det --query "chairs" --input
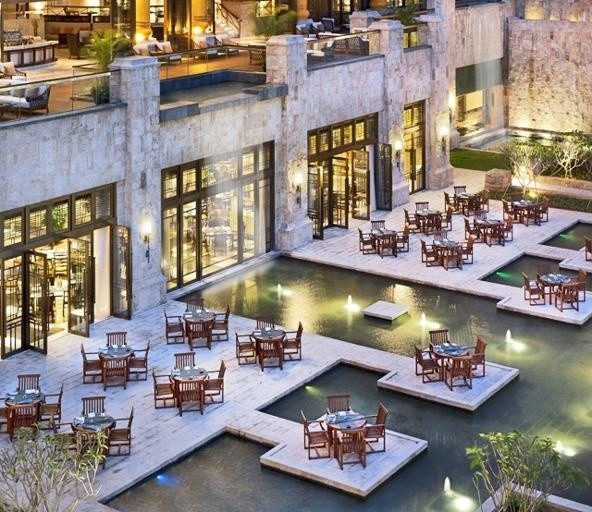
[133,31,240,64]
[296,17,350,41]
[44,24,94,59]
[357,186,592,312]
[248,45,266,68]
[6,250,81,323]
[0,62,52,121]
